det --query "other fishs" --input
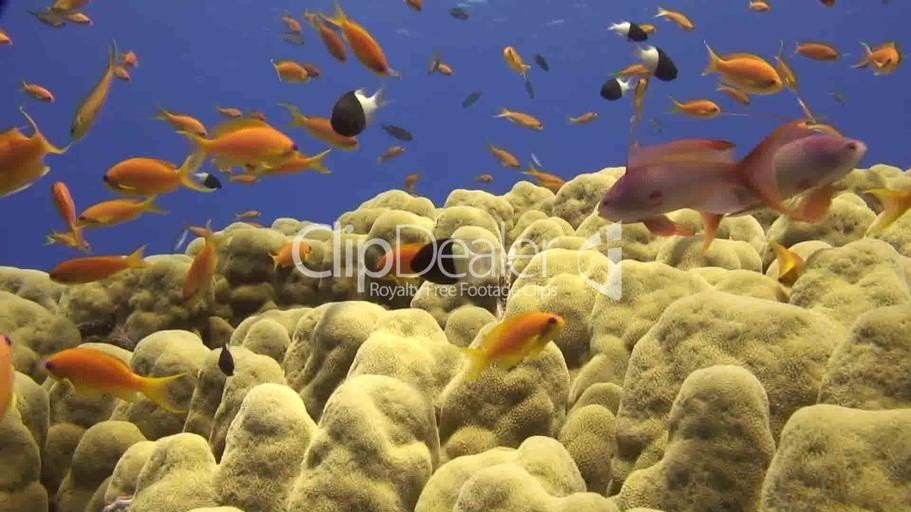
[1,1,907,425]
